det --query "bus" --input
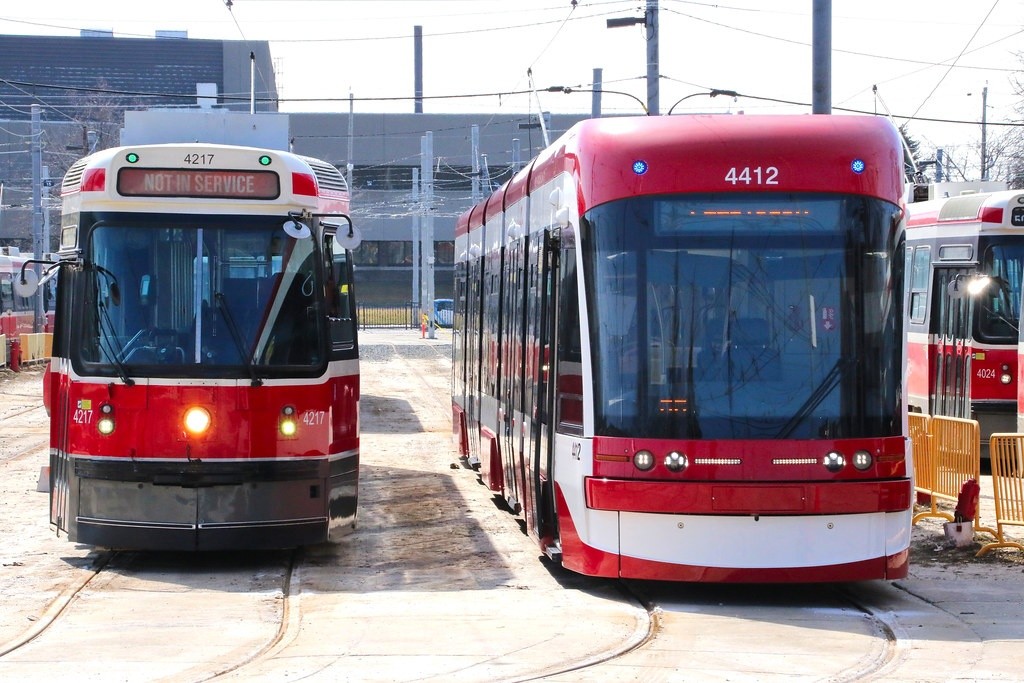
[12,142,363,555]
[900,181,1024,470]
[451,113,915,592]
[0,244,60,338]
